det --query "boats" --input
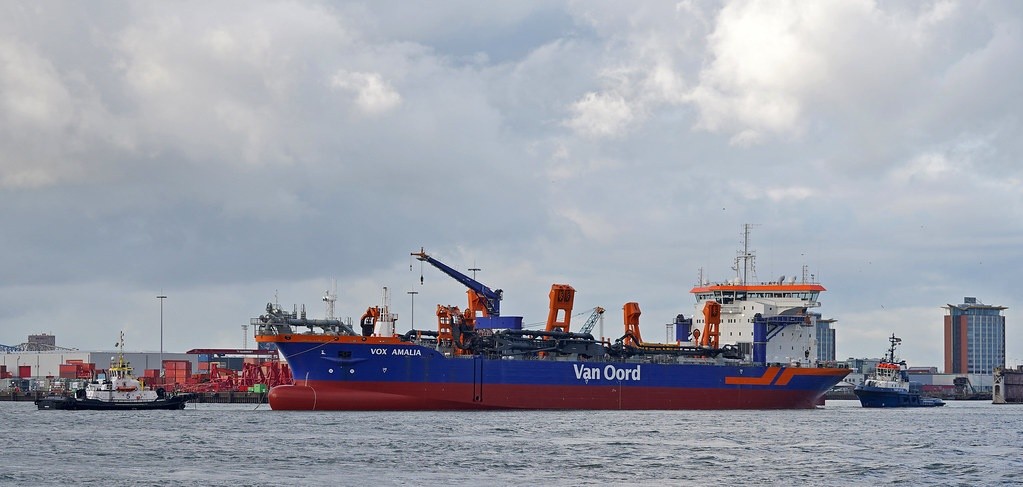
[36,353,197,411]
[254,245,853,412]
[853,333,946,408]
